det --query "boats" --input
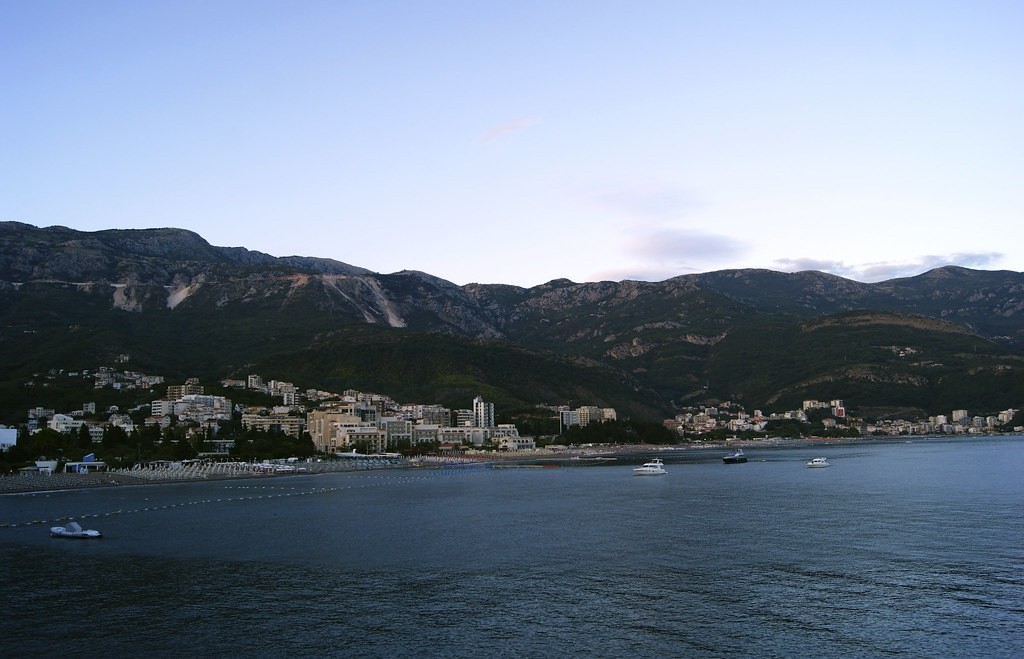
[632,458,668,476]
[50,521,102,538]
[721,452,747,463]
[805,457,830,468]
